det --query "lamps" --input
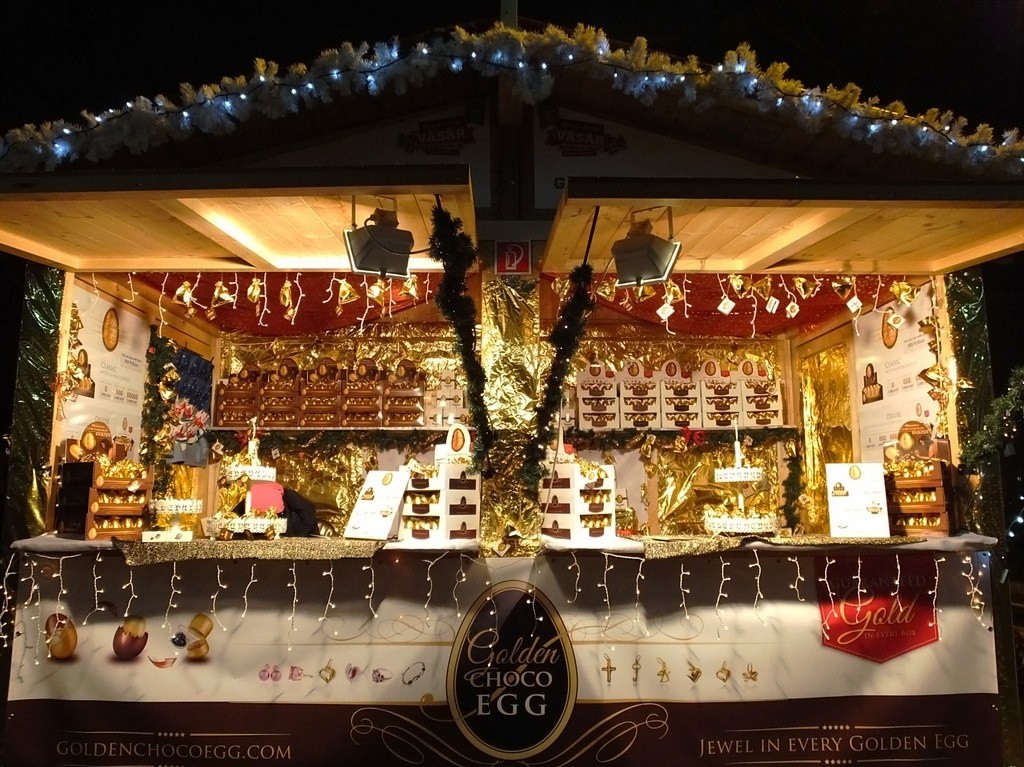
[342,194,413,279]
[611,207,682,287]
